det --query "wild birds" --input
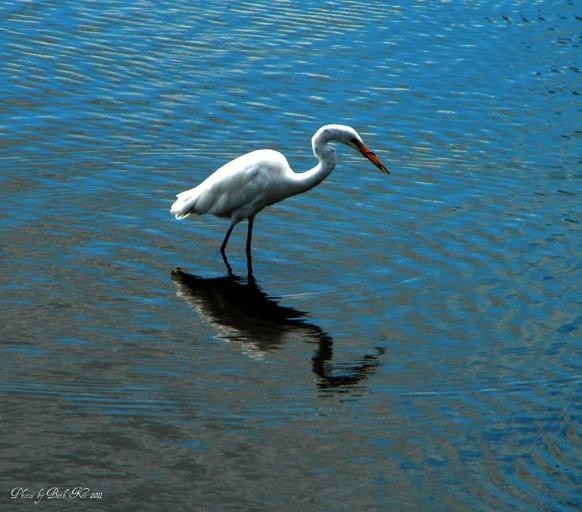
[169,253,382,393]
[173,122,389,253]
